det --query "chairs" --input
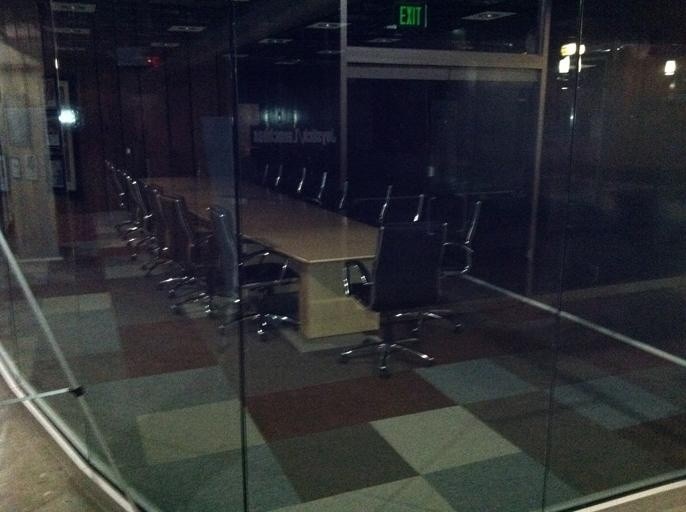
[104,154,479,340]
[338,222,449,372]
